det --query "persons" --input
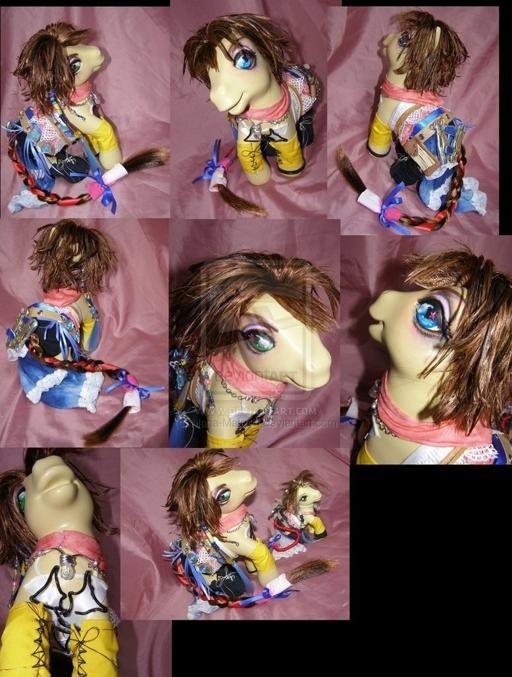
[337,12,487,235]
